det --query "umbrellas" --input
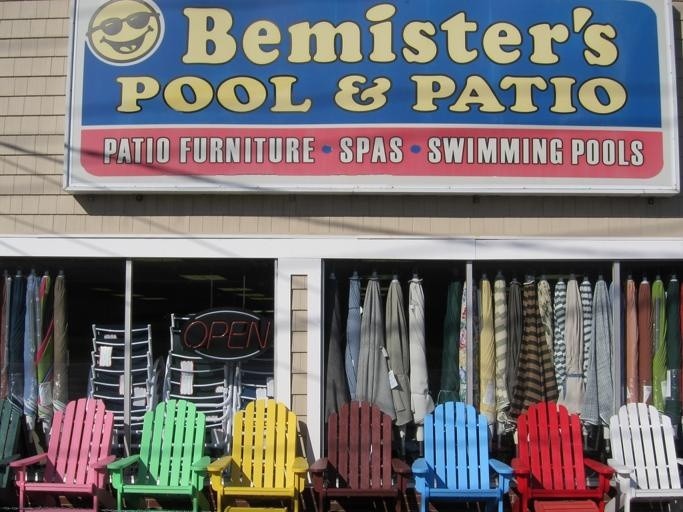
[1,266,70,455]
